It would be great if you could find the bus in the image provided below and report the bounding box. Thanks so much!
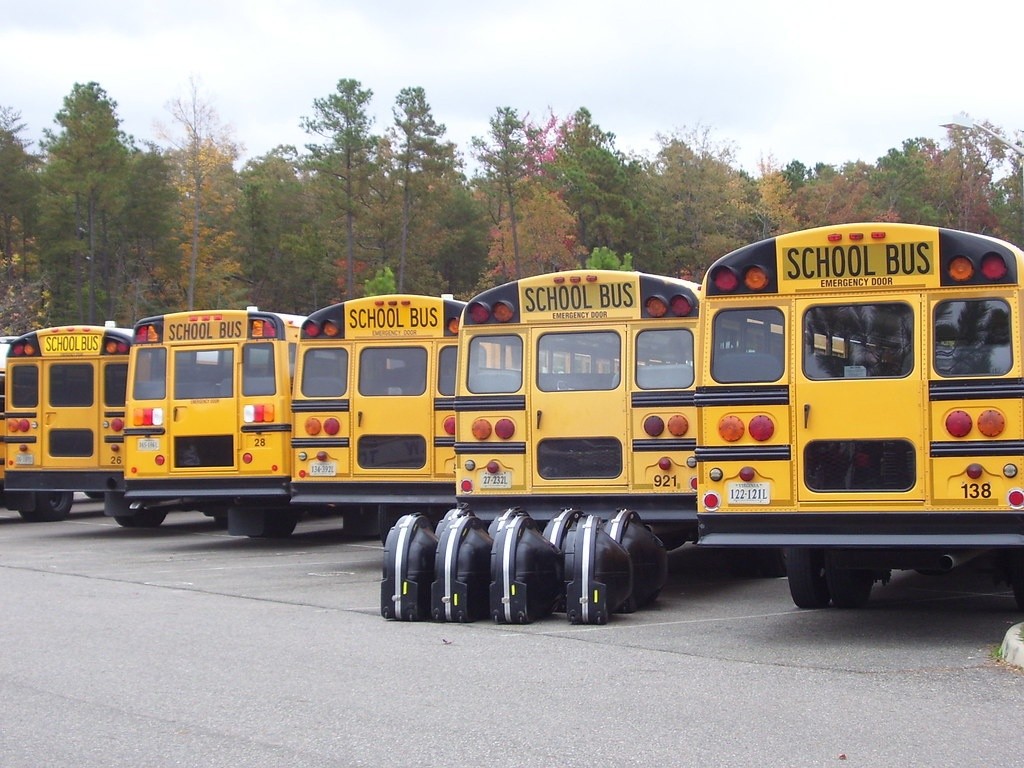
[0,320,136,526]
[289,294,467,545]
[695,221,1024,607]
[123,306,309,539]
[452,270,749,573]
